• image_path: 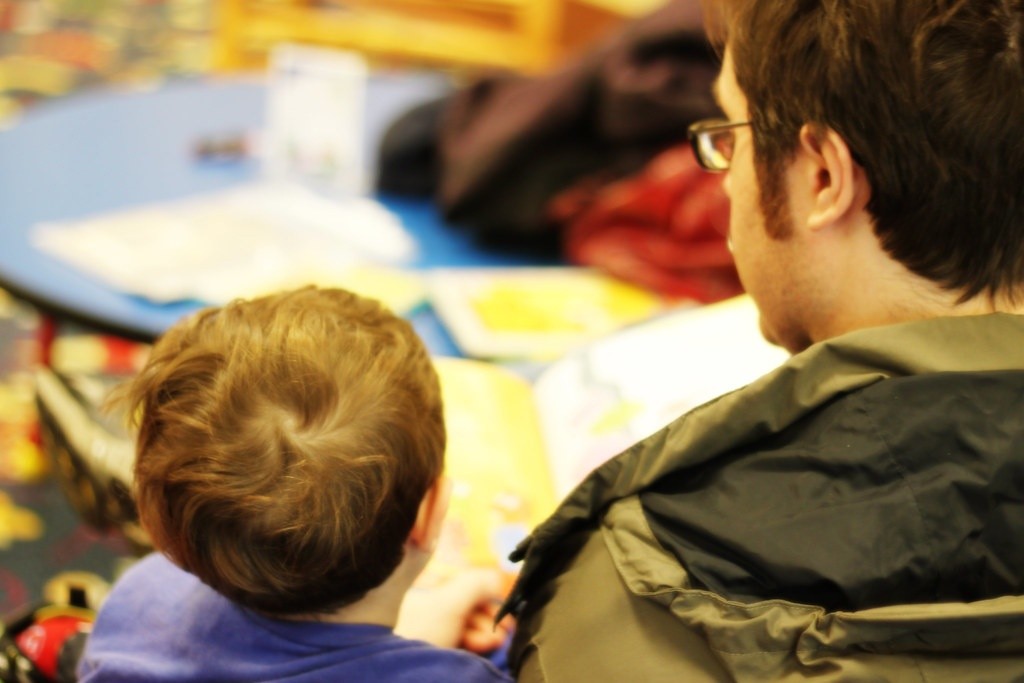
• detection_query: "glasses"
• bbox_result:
[688,117,751,172]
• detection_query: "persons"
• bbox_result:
[75,283,524,683]
[487,0,1024,683]
